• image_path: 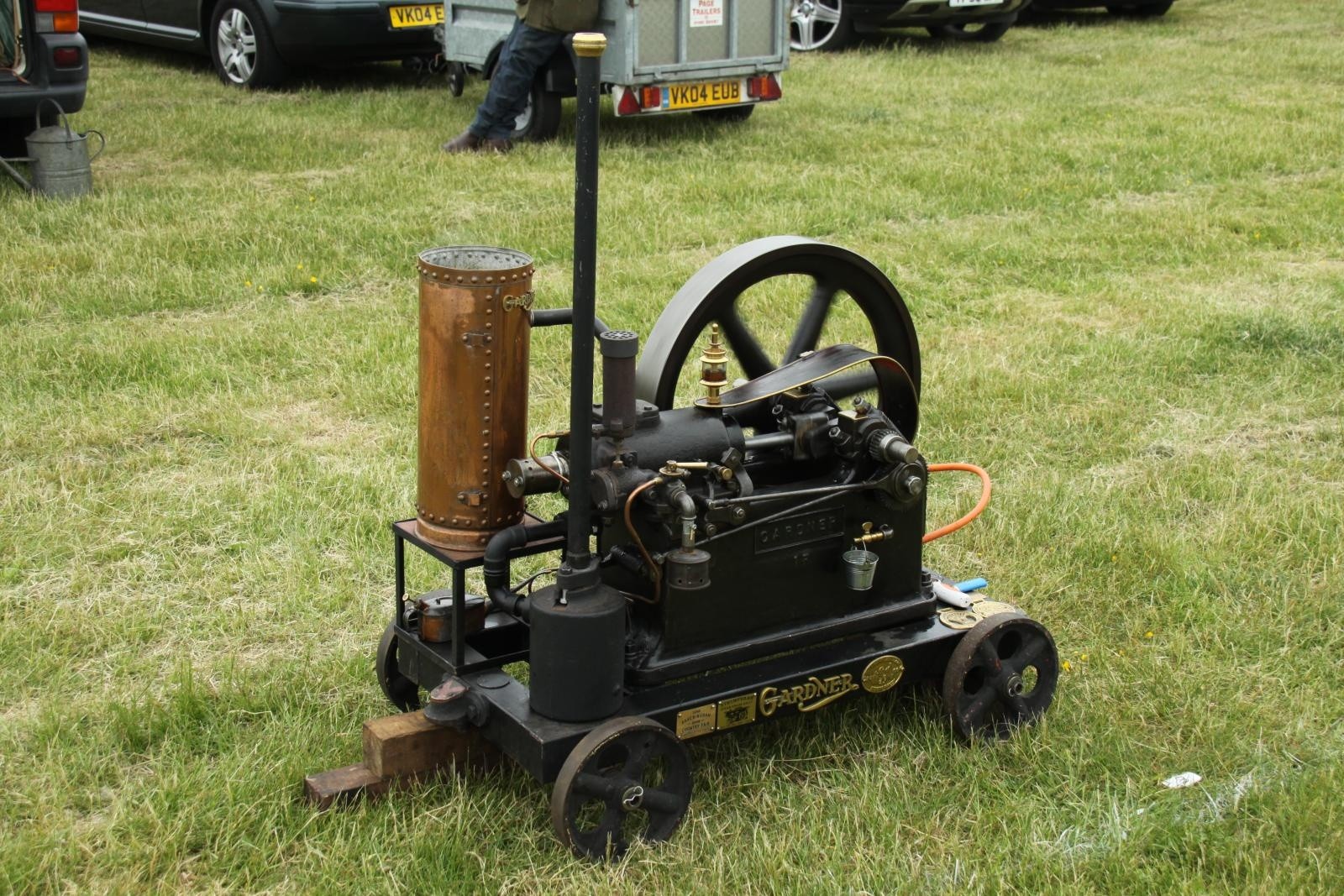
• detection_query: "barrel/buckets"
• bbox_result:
[842,537,879,591]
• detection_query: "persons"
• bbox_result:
[442,0,600,155]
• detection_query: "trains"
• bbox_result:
[374,26,1060,867]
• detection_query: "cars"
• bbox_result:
[0,0,89,154]
[78,0,449,92]
[789,0,1028,55]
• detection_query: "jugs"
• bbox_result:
[1,97,106,197]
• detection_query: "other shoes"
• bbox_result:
[482,137,513,152]
[444,131,478,152]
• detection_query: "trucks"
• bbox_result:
[445,0,792,126]
